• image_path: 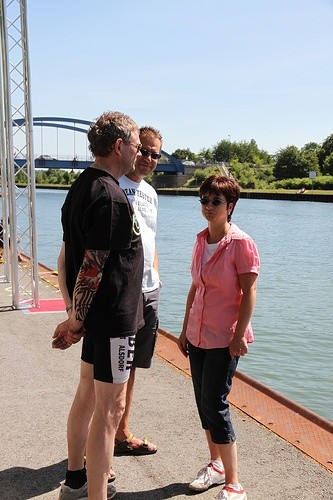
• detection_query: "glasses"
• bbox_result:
[127,140,142,152]
[140,148,161,159]
[199,198,228,206]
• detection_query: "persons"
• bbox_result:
[84,128,163,481]
[177,176,260,500]
[51,112,144,499]
[0,216,27,261]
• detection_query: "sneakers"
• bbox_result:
[58,483,116,500]
[189,463,225,491]
[215,483,247,500]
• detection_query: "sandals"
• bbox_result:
[84,456,116,481]
[114,432,157,456]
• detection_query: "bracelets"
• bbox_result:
[66,304,72,312]
